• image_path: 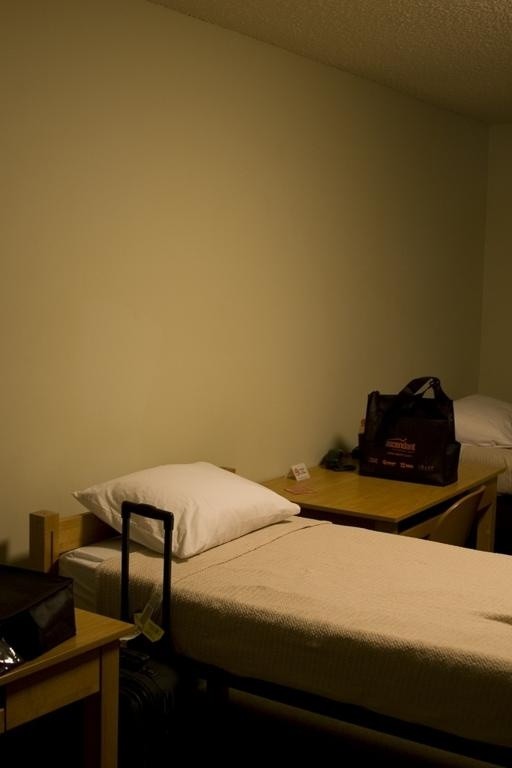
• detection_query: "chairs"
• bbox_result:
[431,485,486,547]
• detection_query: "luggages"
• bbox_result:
[74,501,193,768]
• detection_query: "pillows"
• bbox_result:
[453,394,511,448]
[74,458,301,559]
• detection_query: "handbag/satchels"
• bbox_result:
[358,377,461,487]
[0,564,76,677]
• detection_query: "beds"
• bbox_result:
[459,443,512,494]
[25,466,512,768]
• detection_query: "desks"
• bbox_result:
[261,460,507,554]
[0,601,136,766]
